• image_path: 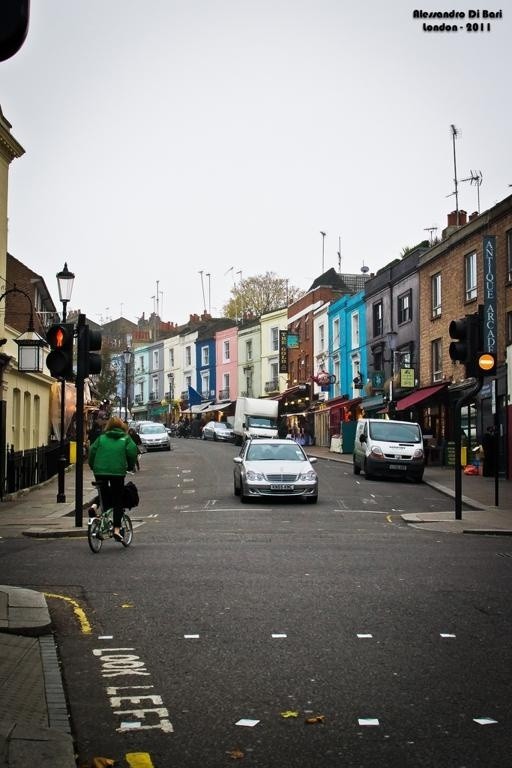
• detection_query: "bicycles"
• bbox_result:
[85,479,136,555]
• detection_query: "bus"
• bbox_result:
[201,420,239,443]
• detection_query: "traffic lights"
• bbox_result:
[44,319,77,383]
[445,315,473,365]
[82,323,106,381]
[476,351,501,378]
[387,400,399,417]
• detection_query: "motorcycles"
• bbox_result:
[170,420,202,439]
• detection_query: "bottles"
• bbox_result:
[106,515,113,529]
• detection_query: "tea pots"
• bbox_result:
[310,370,336,387]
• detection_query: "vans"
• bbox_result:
[353,418,429,483]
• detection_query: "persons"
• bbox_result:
[126,427,141,476]
[481,425,497,478]
[87,414,139,542]
[171,414,203,440]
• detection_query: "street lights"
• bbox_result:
[122,345,134,425]
[53,260,78,506]
[243,365,253,397]
[166,374,174,428]
[386,326,401,420]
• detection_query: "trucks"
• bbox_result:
[232,396,281,446]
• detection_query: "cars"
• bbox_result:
[111,405,175,452]
[230,436,324,510]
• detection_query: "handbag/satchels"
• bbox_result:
[124,482,139,508]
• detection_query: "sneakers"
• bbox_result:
[114,534,123,539]
[88,508,97,517]
